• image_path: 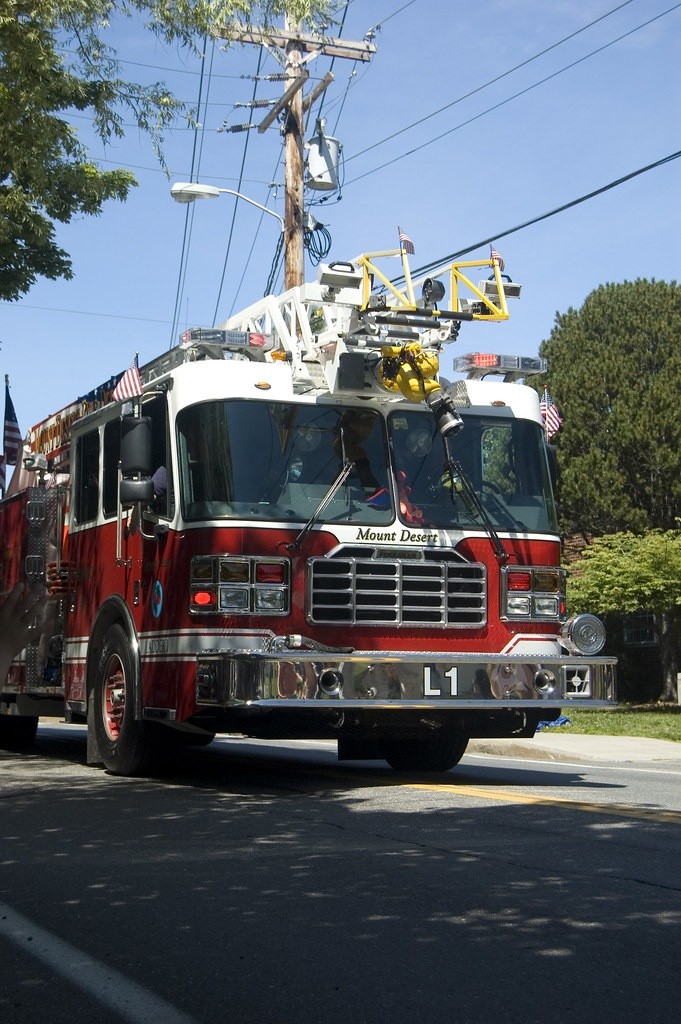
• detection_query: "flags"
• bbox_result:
[107,356,149,402]
[539,390,563,438]
[0,455,6,489]
[2,380,23,465]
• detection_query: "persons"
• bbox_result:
[0,581,55,693]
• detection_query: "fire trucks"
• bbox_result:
[2,229,608,778]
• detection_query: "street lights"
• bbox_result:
[170,182,305,292]
